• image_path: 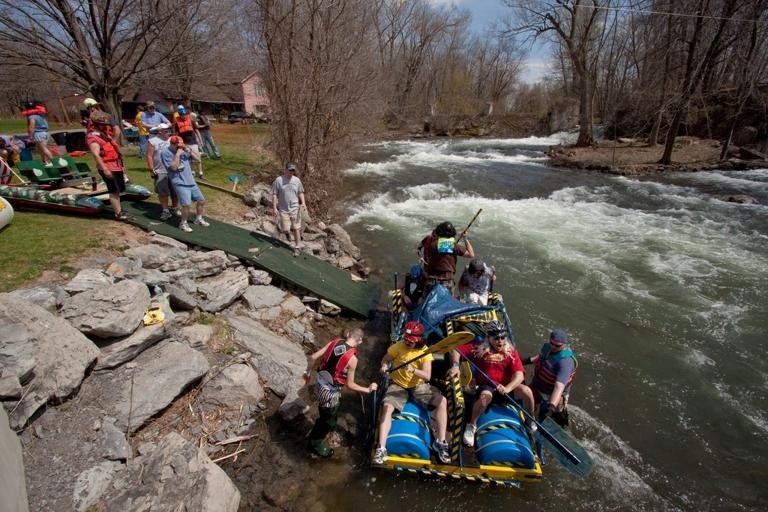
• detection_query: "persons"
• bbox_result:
[79,98,120,142]
[86,111,135,220]
[306,327,377,457]
[459,260,496,306]
[400,264,426,310]
[417,228,435,270]
[172,106,197,122]
[161,137,210,232]
[135,106,148,158]
[25,103,54,166]
[174,108,204,179]
[421,222,475,304]
[141,101,172,138]
[372,322,451,465]
[197,109,221,158]
[147,124,182,220]
[522,328,578,430]
[444,321,536,447]
[272,163,306,255]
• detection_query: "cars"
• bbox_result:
[228,111,248,124]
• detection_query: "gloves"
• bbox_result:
[519,356,532,365]
[539,403,554,421]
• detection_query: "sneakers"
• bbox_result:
[179,219,192,233]
[199,174,206,179]
[116,211,130,220]
[433,438,451,464]
[464,424,478,447]
[215,154,221,158]
[194,219,210,227]
[171,207,182,215]
[159,210,172,221]
[42,160,53,167]
[294,246,300,258]
[527,416,538,430]
[372,446,390,464]
[287,241,296,249]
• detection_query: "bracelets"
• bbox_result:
[412,368,416,374]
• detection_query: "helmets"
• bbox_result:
[177,105,184,109]
[409,265,422,277]
[486,320,508,338]
[179,109,186,114]
[401,322,425,344]
[92,111,116,125]
[436,221,457,238]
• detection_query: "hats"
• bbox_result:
[156,124,170,134]
[286,162,297,170]
[80,98,103,109]
[197,109,204,114]
[25,101,33,108]
[469,258,485,273]
[171,136,184,146]
[144,101,156,109]
[551,329,568,346]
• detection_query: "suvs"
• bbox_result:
[80,101,170,126]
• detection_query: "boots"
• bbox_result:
[308,422,336,457]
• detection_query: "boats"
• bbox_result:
[371,288,545,487]
[0,158,152,213]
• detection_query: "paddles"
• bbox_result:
[230,175,241,192]
[389,331,474,374]
[420,316,594,478]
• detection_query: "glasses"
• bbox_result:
[492,336,506,342]
[550,341,565,348]
